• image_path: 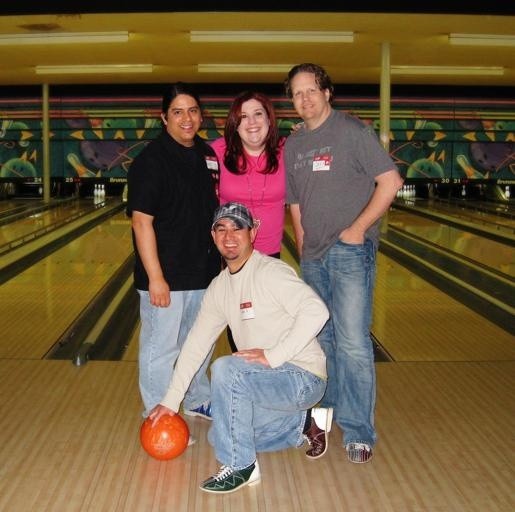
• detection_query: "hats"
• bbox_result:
[211,202,253,230]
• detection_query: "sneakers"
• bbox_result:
[184,399,212,420]
[199,459,261,494]
[187,436,197,446]
[347,443,374,464]
[302,407,333,459]
